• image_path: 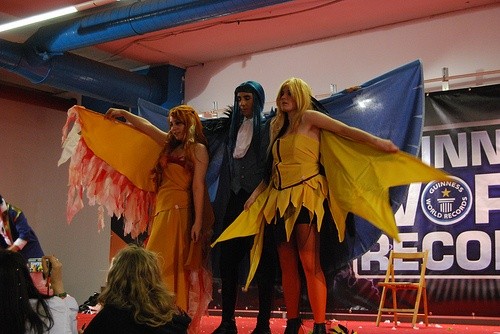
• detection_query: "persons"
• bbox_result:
[211,78,400,334]
[0,195,45,263]
[103,105,213,334]
[203,80,362,334]
[78,243,192,334]
[0,249,80,334]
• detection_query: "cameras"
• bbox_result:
[27,258,51,272]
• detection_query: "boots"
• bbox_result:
[212,277,238,334]
[251,288,274,334]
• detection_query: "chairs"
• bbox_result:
[375,250,430,328]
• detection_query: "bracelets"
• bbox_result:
[12,245,20,251]
[54,292,66,297]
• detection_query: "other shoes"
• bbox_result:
[284,317,307,334]
[313,323,326,334]
